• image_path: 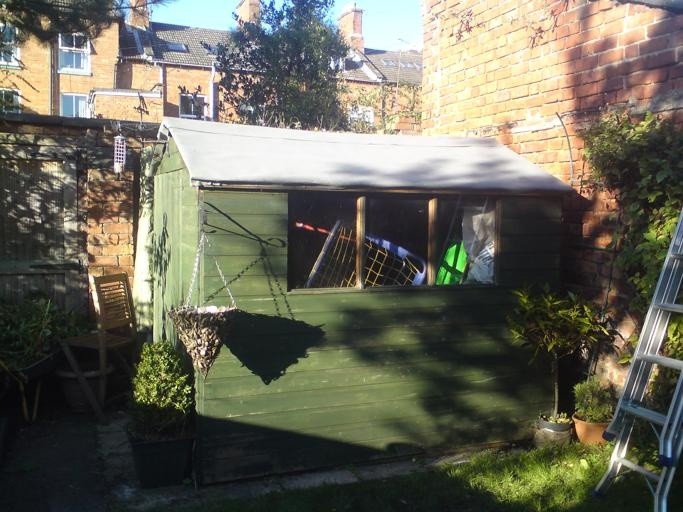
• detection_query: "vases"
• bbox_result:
[58,365,108,417]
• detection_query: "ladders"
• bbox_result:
[590,204,683,511]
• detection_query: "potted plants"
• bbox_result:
[505,280,618,449]
[124,338,196,490]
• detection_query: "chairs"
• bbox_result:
[60,270,141,419]
